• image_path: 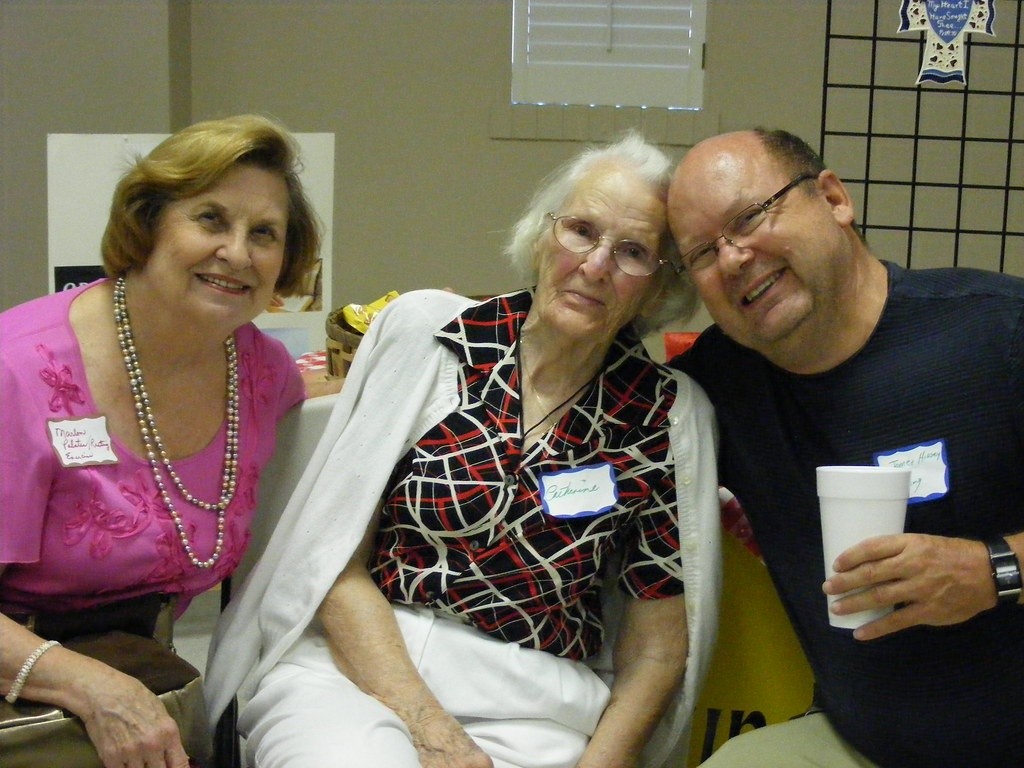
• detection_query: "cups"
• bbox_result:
[815,463,913,631]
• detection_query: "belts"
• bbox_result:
[4,595,170,642]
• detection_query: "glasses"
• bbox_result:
[548,212,673,277]
[673,171,817,275]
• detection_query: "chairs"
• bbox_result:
[220,394,350,767]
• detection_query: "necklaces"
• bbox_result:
[115,274,239,567]
[517,329,599,448]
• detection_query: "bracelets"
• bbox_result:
[4,640,62,704]
[981,535,1022,611]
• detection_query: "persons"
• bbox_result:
[0,114,328,768]
[202,134,716,768]
[662,125,1024,768]
